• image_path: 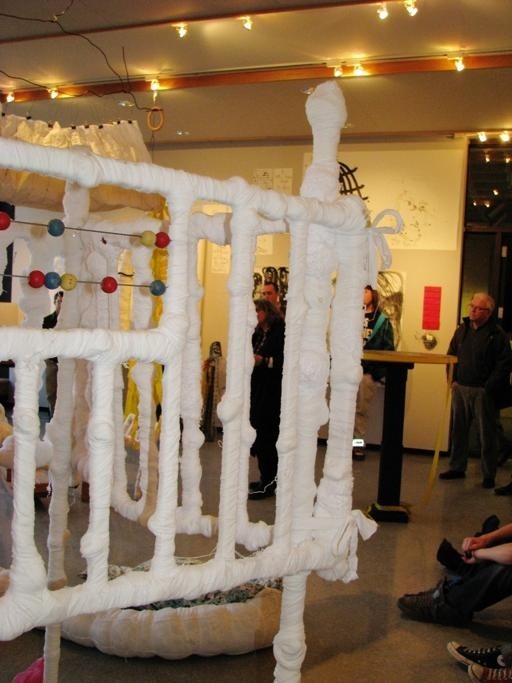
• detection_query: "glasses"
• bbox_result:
[469,304,489,311]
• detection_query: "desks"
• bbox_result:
[352,350,459,524]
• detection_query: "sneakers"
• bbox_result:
[467,663,512,683]
[397,576,473,625]
[447,640,506,668]
[482,478,494,488]
[439,469,465,479]
[495,482,512,496]
[248,481,276,499]
[353,447,365,460]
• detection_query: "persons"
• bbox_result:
[41,291,64,410]
[353,284,396,459]
[396,522,512,627]
[437,291,511,489]
[446,639,512,682]
[247,298,286,501]
[255,282,286,322]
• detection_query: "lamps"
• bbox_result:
[6,0,465,103]
